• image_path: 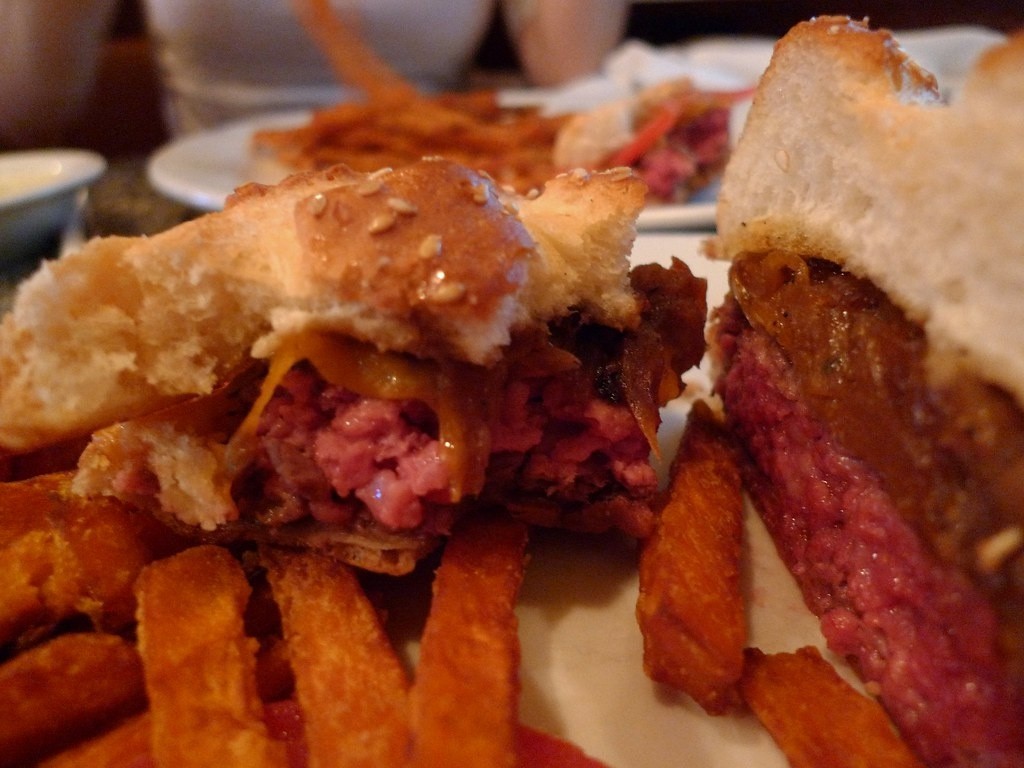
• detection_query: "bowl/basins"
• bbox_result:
[0,147,109,262]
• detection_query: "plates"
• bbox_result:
[395,232,879,768]
[148,85,754,232]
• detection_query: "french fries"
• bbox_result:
[248,0,569,195]
[0,410,945,768]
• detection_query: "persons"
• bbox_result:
[0,0,632,154]
